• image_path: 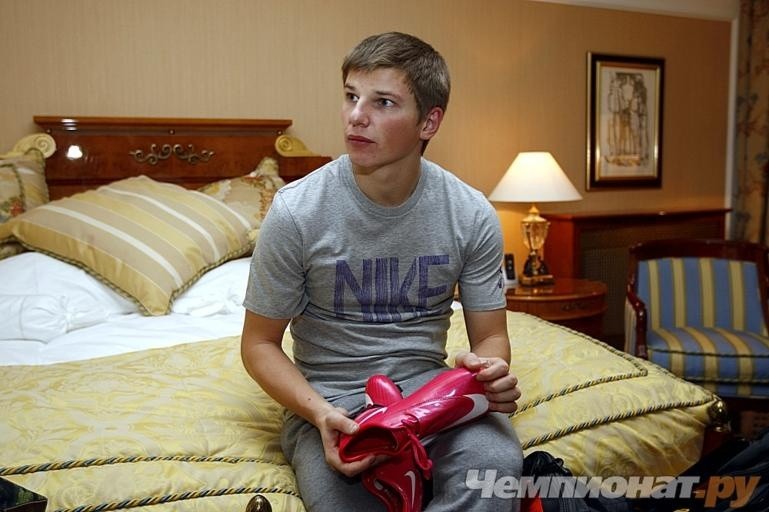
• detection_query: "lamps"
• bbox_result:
[488,152,583,288]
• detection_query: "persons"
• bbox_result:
[237,30,525,511]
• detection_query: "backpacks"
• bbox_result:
[520,451,572,512]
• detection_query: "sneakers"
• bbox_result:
[339,368,491,512]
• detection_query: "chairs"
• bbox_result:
[621,239,769,437]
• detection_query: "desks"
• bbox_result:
[539,208,734,338]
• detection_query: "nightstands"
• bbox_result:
[501,277,606,345]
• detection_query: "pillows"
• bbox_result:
[195,156,287,252]
[11,174,255,317]
[0,253,140,345]
[0,148,49,263]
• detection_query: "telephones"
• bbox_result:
[501,253,519,286]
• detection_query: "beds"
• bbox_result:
[0,115,733,512]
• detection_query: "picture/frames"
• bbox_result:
[587,51,666,190]
